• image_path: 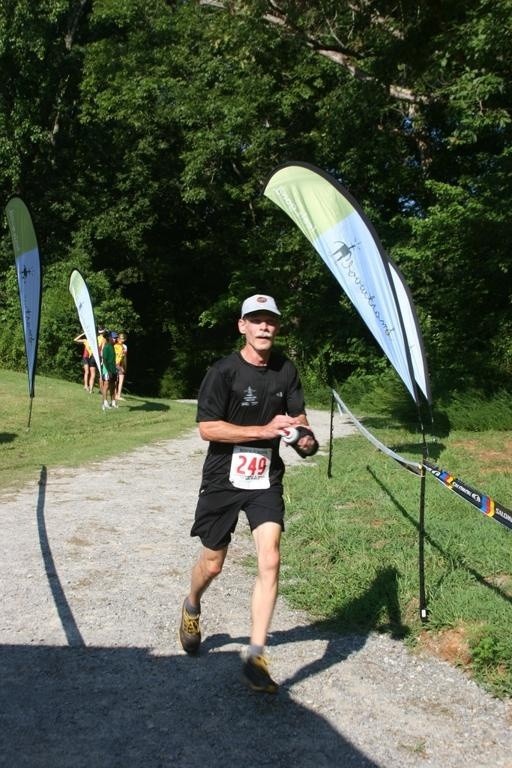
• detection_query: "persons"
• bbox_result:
[74,326,129,409]
[179,294,319,693]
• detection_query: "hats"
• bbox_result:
[241,294,281,316]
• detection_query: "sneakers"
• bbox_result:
[178,598,200,653]
[241,655,278,693]
[103,404,118,409]
